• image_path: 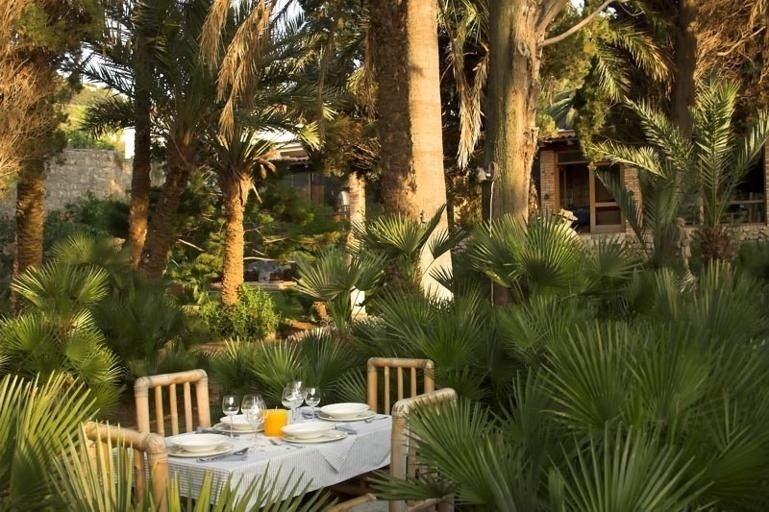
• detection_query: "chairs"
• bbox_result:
[364,356,434,420]
[133,368,211,441]
[332,386,461,510]
[81,420,177,512]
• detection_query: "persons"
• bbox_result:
[670,216,692,276]
[548,208,580,241]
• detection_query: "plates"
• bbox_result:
[281,430,348,444]
[161,414,265,457]
[320,410,377,422]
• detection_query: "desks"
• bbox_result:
[107,407,412,512]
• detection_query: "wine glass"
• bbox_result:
[222,394,267,456]
[281,380,322,423]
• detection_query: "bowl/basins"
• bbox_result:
[321,403,369,418]
[282,422,335,438]
